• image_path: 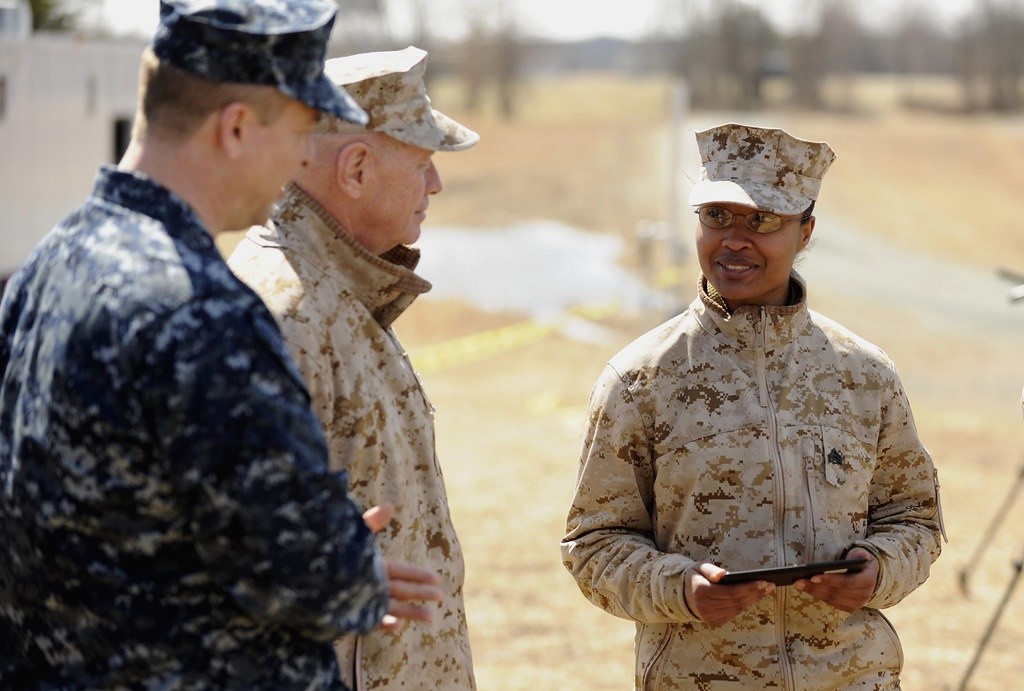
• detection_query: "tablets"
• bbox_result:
[717,558,869,590]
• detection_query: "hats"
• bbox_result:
[325,45,481,151]
[689,123,837,216]
[152,0,371,126]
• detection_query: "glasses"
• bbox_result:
[695,205,813,234]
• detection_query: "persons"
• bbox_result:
[223,46,481,691]
[0,0,443,691]
[559,124,947,691]
